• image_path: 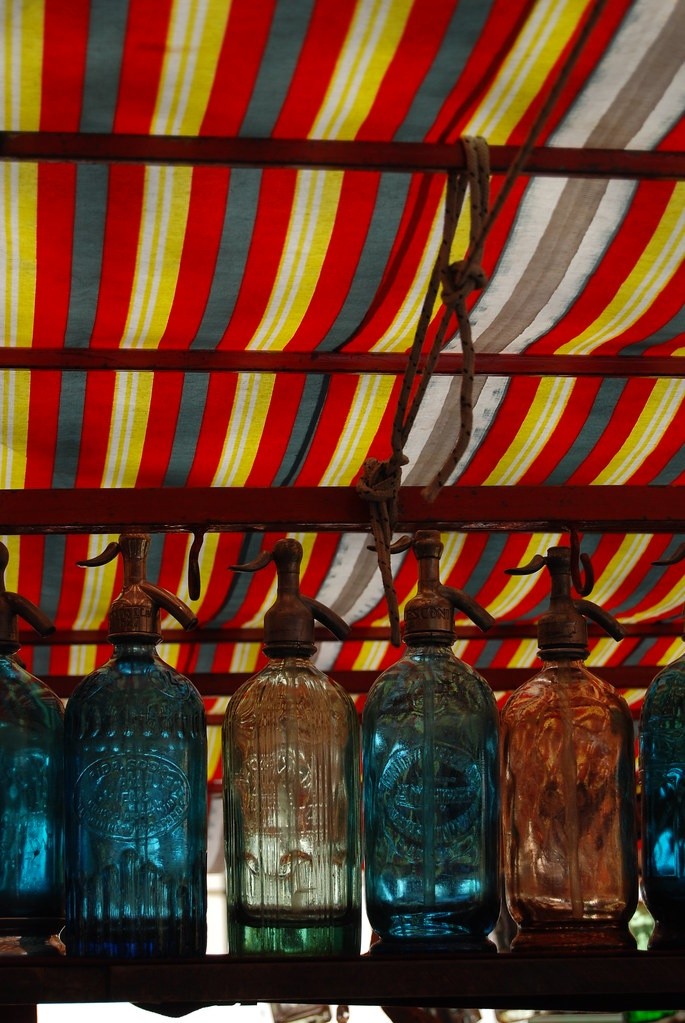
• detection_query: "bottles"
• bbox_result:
[503,649,634,926]
[64,638,208,924]
[221,645,360,922]
[354,631,503,939]
[0,639,64,917]
[640,631,685,923]
